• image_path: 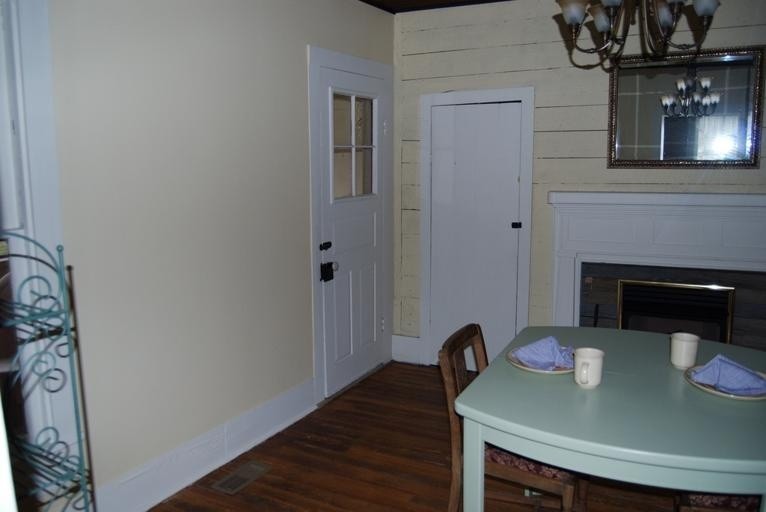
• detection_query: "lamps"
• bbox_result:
[553,0,721,72]
[658,65,719,117]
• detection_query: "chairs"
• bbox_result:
[439,322,589,512]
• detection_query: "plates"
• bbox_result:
[506,346,574,376]
[684,362,765,400]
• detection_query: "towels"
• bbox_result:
[512,335,573,370]
[690,355,766,394]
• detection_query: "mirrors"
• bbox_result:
[607,44,766,168]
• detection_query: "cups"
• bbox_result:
[572,347,606,390]
[669,330,700,372]
